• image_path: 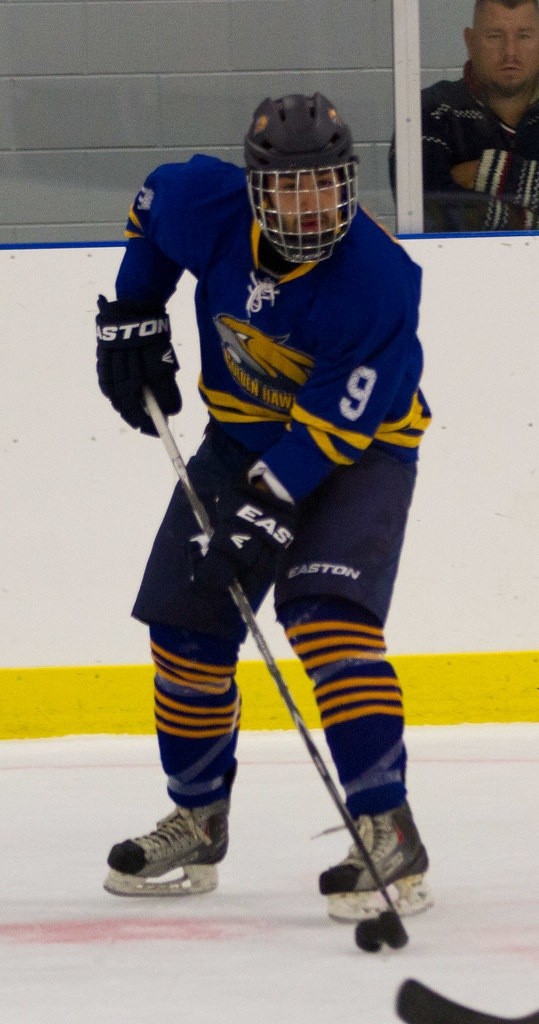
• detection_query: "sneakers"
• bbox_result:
[320,798,432,919]
[102,794,231,893]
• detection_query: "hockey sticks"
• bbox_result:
[96,293,410,952]
[393,977,539,1024]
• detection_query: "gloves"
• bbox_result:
[96,295,183,437]
[187,482,302,590]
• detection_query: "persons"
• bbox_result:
[386,1,539,233]
[95,92,435,924]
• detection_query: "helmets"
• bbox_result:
[243,94,359,264]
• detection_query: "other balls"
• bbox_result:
[355,919,384,951]
